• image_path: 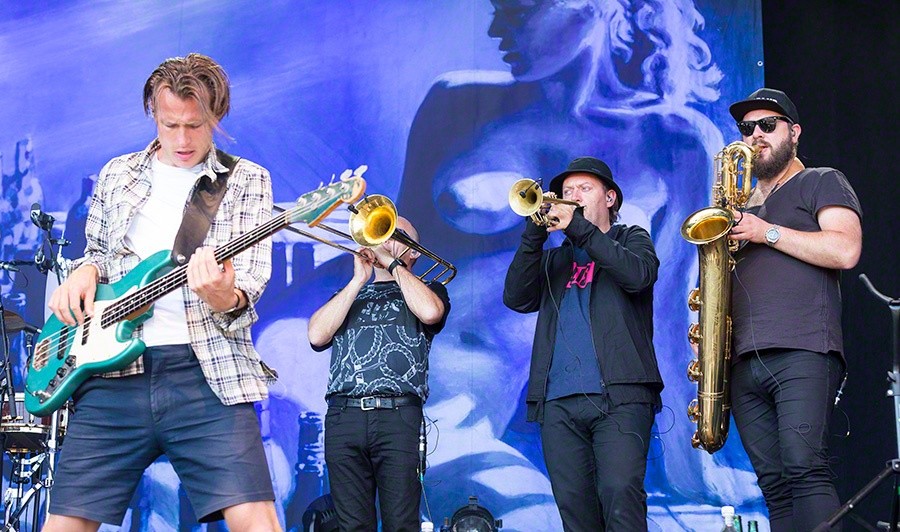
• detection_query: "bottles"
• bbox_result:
[282,411,330,532]
[720,504,759,532]
[441,517,451,532]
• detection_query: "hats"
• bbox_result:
[549,157,623,213]
[728,88,799,124]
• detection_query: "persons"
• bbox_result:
[502,157,665,532]
[41,52,285,532]
[307,214,452,532]
[716,87,862,532]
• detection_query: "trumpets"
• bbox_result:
[509,177,584,228]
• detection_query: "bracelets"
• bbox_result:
[222,293,240,314]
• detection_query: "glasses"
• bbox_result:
[736,116,795,137]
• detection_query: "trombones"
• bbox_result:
[270,192,458,288]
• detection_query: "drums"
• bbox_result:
[0,391,72,449]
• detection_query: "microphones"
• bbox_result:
[348,205,358,214]
[520,178,543,199]
[0,261,20,272]
[721,197,743,226]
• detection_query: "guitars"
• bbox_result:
[23,161,370,420]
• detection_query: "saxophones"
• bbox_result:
[685,139,765,455]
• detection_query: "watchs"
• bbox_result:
[387,258,408,276]
[764,223,782,248]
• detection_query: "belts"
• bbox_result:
[329,396,421,410]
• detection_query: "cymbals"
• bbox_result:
[1,310,25,335]
